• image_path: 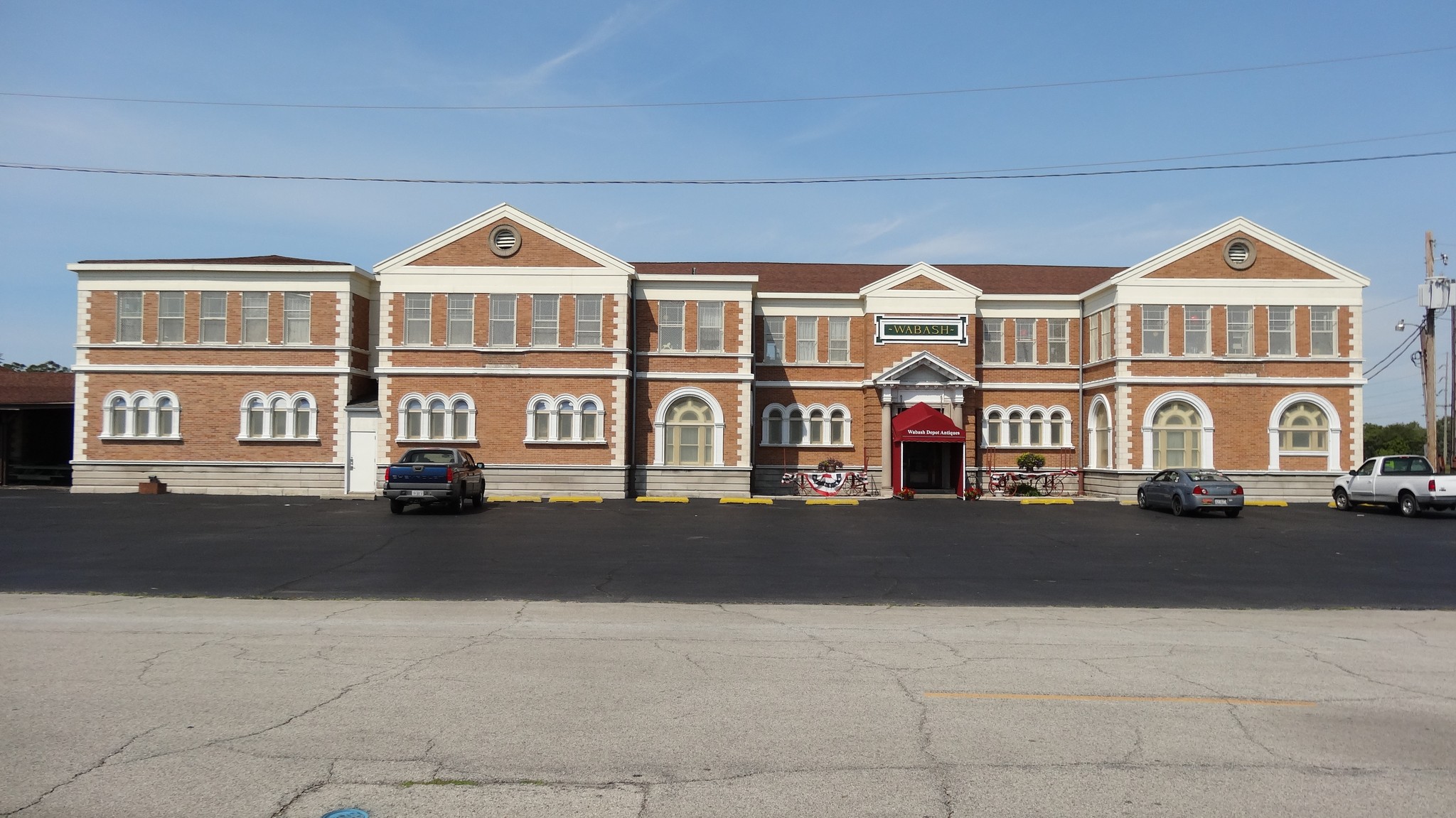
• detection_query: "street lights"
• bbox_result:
[1396,320,1439,472]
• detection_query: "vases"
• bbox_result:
[903,496,909,501]
[969,496,975,502]
[994,491,1005,496]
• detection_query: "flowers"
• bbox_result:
[898,487,917,496]
[964,487,984,498]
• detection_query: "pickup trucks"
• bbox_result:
[1330,455,1456,518]
[383,449,486,514]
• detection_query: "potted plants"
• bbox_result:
[140,476,167,494]
[1016,452,1046,473]
[816,457,844,472]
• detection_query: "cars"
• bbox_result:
[1137,468,1245,517]
